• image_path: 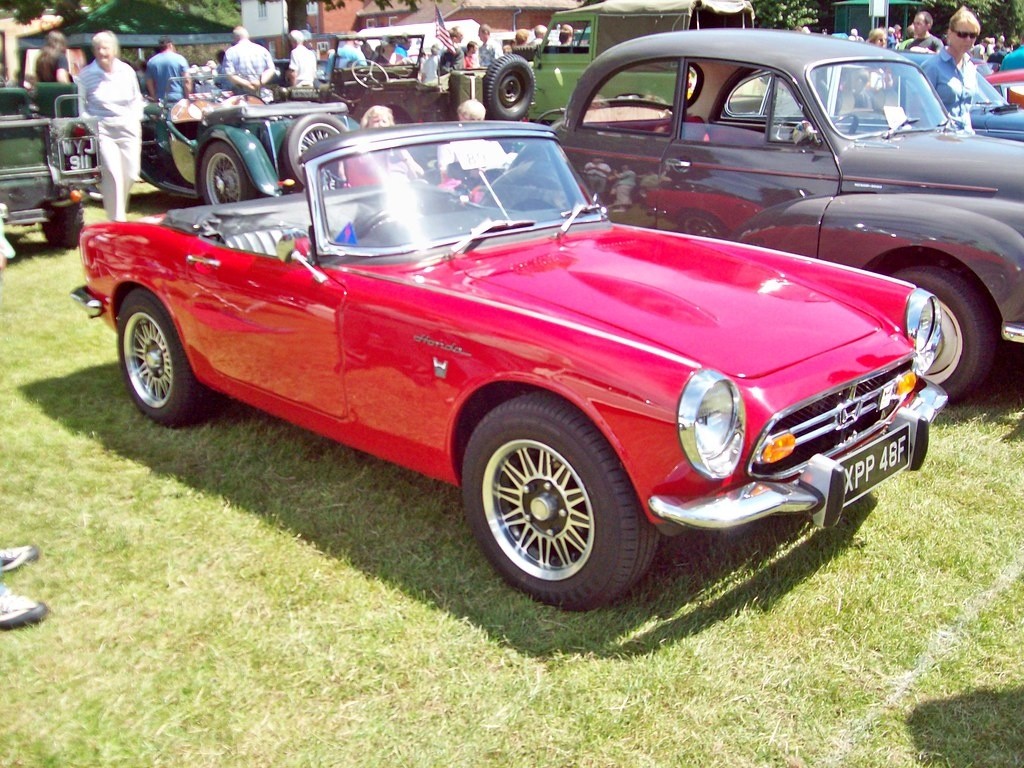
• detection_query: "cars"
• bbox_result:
[901,54,1024,140]
[555,28,1024,403]
[319,55,536,121]
[140,73,349,205]
[0,88,85,250]
[69,121,948,612]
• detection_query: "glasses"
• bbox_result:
[878,39,883,42]
[951,29,978,39]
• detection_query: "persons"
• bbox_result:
[346,105,426,188]
[139,22,575,104]
[0,543,46,631]
[33,29,72,84]
[579,156,677,213]
[76,30,145,225]
[436,97,518,204]
[795,6,1024,139]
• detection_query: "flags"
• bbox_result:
[435,4,456,54]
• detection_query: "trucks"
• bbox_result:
[535,1,756,119]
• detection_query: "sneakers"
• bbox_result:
[0,544,39,573]
[0,584,49,632]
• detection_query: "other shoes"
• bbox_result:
[613,208,627,213]
